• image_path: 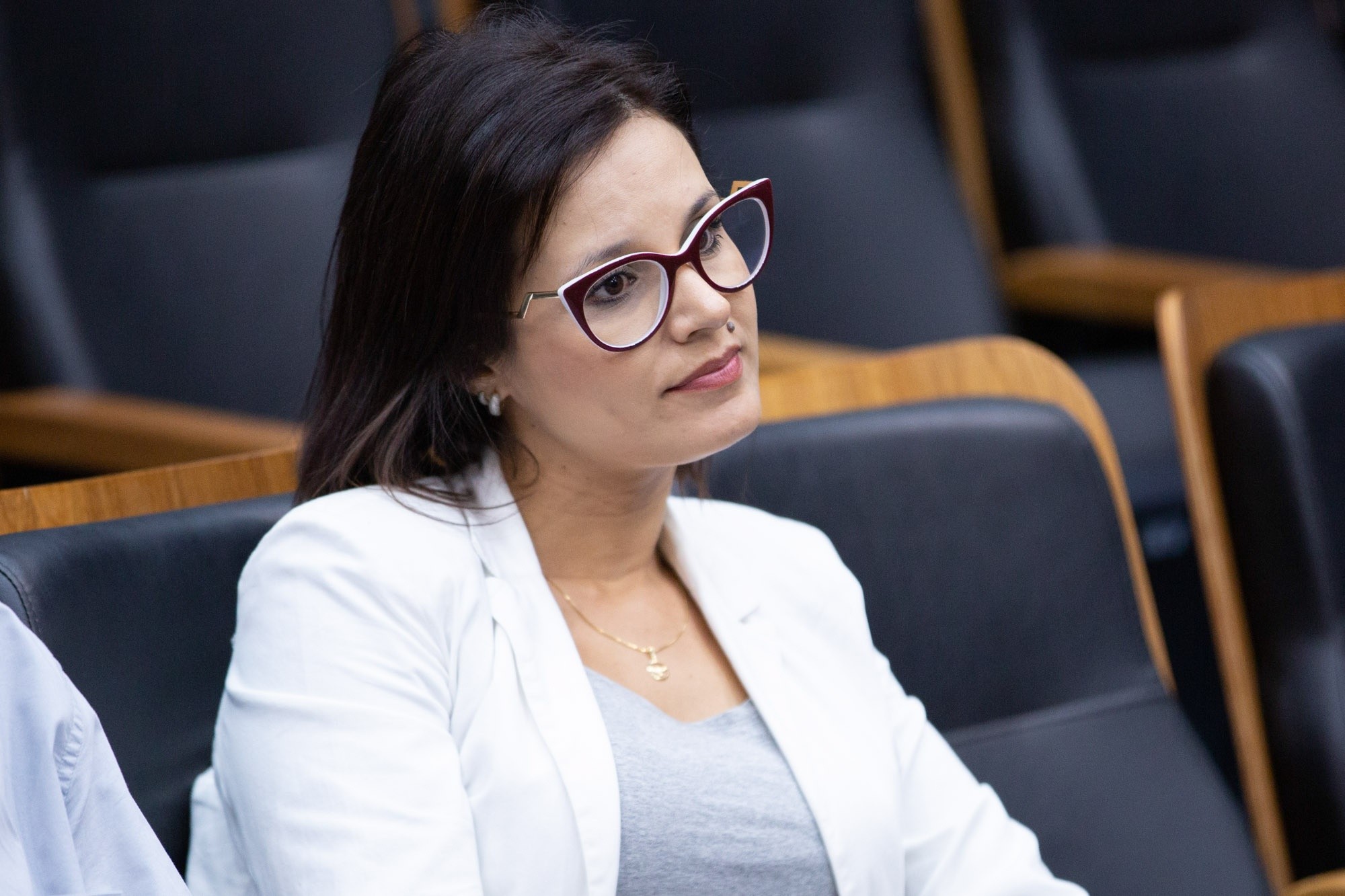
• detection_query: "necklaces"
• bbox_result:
[545,576,689,681]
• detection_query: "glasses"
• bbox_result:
[506,178,775,352]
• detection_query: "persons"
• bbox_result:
[0,604,192,896]
[187,17,1090,896]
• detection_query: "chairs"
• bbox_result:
[0,0,1345,896]
